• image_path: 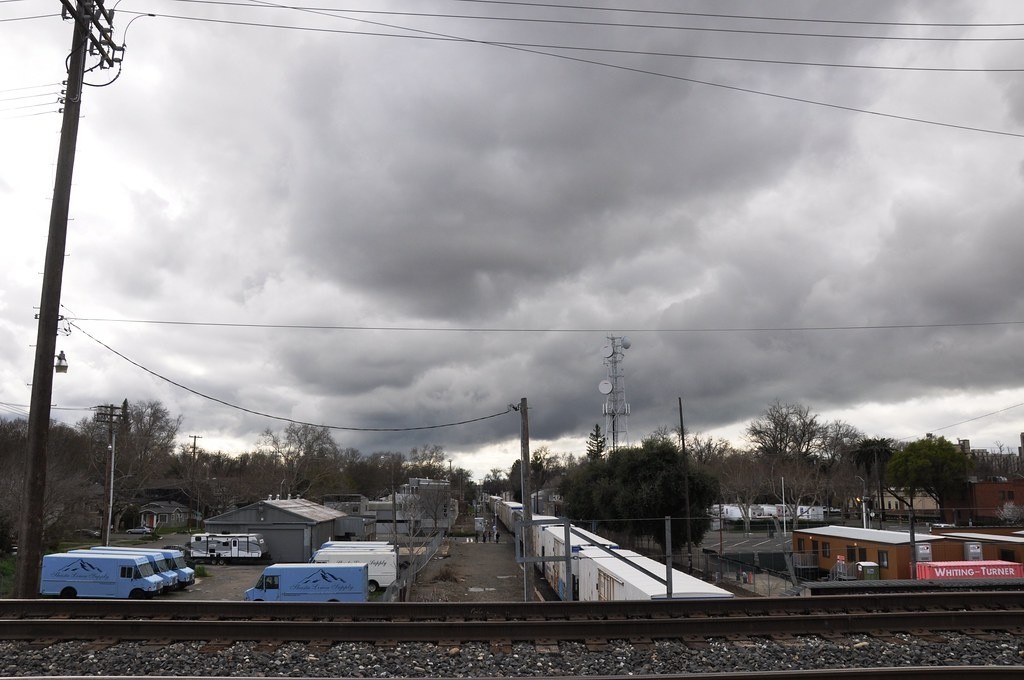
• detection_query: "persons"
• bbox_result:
[488,531,492,543]
[475,530,480,543]
[492,524,500,543]
[482,531,487,543]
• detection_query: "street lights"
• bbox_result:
[105,436,116,545]
[101,474,134,546]
[195,477,217,532]
[18,350,68,598]
[448,458,453,499]
[380,454,399,583]
[494,501,500,528]
[854,476,867,528]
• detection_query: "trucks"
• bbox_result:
[38,552,164,600]
[307,540,400,593]
[67,548,180,595]
[90,546,196,591]
[243,562,370,601]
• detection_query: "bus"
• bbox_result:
[189,531,270,565]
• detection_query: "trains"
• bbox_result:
[488,495,736,602]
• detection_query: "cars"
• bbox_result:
[126,526,152,535]
[71,529,103,540]
